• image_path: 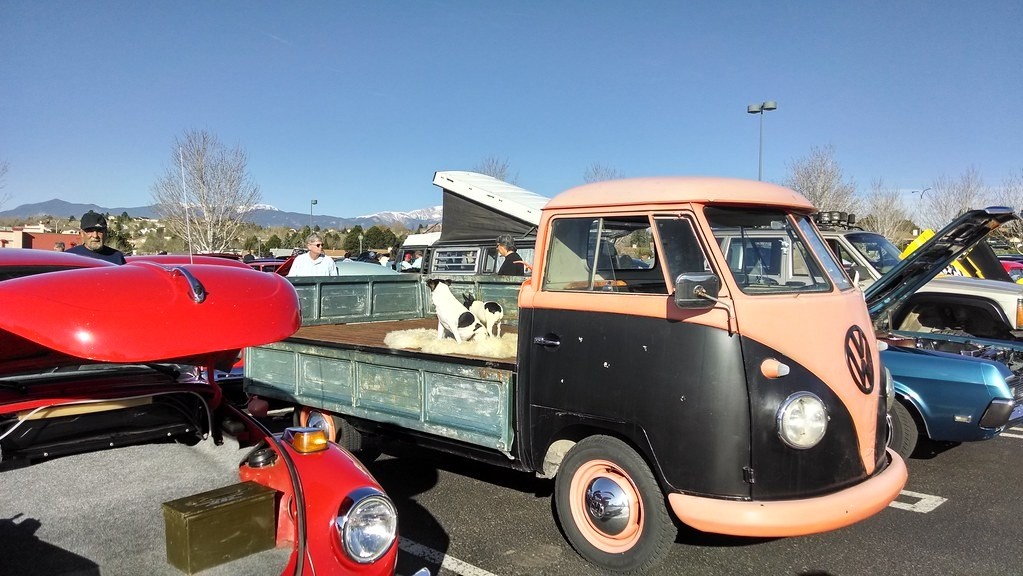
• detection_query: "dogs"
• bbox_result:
[426,279,503,342]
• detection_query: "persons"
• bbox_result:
[286,234,337,276]
[400,251,422,270]
[53,211,127,264]
[496,234,524,275]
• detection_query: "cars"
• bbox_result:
[898,229,1023,286]
[1,250,400,576]
[861,206,1023,461]
[121,256,250,390]
[246,261,285,273]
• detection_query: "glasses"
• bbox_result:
[310,244,323,247]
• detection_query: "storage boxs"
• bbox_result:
[162,480,277,576]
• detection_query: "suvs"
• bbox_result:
[709,212,1023,348]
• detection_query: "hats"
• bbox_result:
[406,254,412,258]
[415,251,423,256]
[80,210,107,231]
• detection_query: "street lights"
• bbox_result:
[311,199,317,234]
[748,103,778,179]
[912,186,933,236]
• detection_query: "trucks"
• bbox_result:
[393,171,537,276]
[241,175,909,575]
